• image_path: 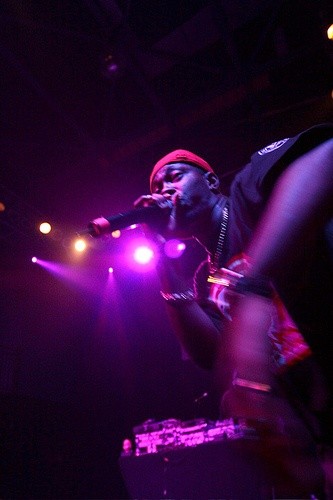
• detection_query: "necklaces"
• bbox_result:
[206,206,232,277]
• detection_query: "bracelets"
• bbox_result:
[158,288,199,303]
[218,265,274,302]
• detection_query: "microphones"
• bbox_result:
[194,393,208,402]
[88,201,177,239]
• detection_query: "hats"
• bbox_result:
[149,149,214,195]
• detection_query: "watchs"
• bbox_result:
[140,122,332,500]
